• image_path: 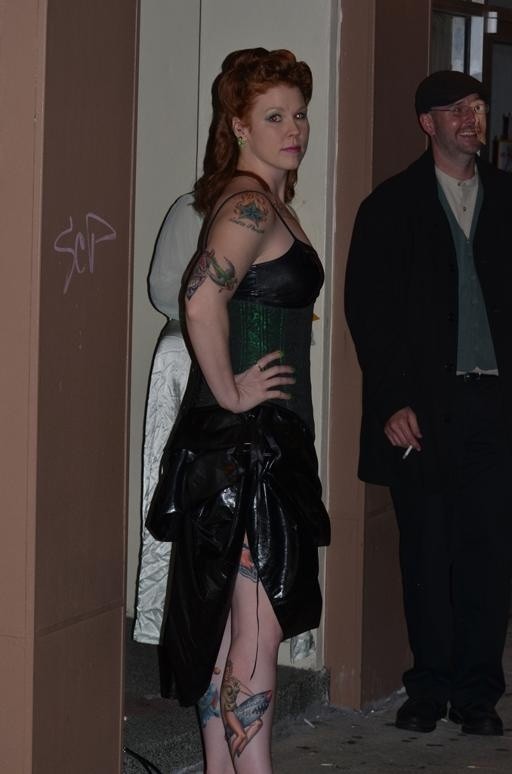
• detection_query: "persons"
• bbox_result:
[146,47,332,773]
[345,69,512,736]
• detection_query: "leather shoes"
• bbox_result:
[395,697,448,733]
[447,701,504,735]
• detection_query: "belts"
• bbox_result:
[456,373,496,383]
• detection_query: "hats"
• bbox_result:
[414,70,487,115]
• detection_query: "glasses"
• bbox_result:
[429,102,490,117]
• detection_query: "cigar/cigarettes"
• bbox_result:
[402,445,413,460]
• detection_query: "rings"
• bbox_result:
[255,363,262,372]
